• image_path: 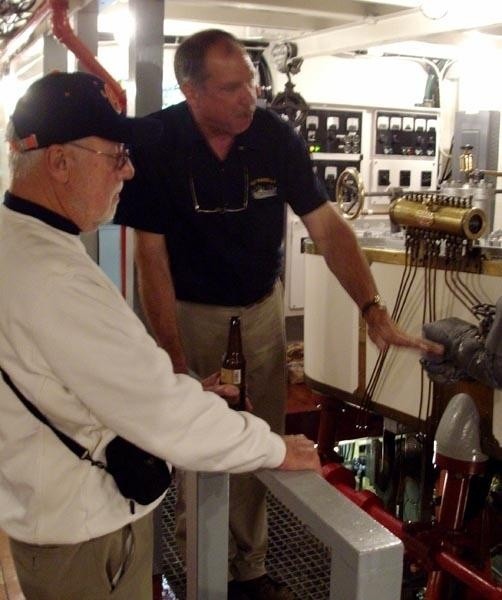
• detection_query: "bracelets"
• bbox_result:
[361,294,386,318]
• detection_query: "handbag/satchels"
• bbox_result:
[104,434,171,505]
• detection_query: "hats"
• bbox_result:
[10,70,163,154]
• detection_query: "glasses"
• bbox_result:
[67,141,129,170]
[188,168,249,215]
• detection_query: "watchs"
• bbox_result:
[111,27,446,600]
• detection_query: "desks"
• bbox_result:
[301,238,502,447]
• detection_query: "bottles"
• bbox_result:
[219,315,246,411]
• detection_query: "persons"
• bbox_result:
[1,64,323,600]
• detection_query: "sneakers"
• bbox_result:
[228,574,295,600]
